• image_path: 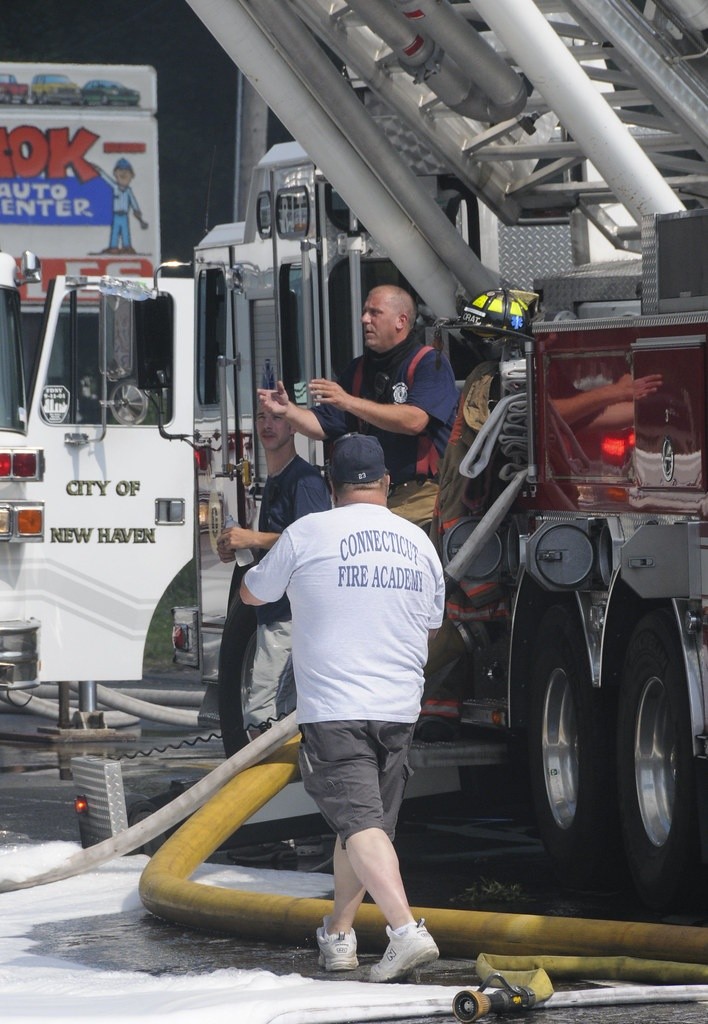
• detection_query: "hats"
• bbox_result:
[330,432,385,484]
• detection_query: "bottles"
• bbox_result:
[224,515,254,567]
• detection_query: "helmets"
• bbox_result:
[459,287,532,338]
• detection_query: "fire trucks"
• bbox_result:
[2,0,708,906]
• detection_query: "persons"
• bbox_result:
[416,337,662,744]
[216,390,446,983]
[253,284,463,528]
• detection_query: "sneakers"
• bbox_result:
[370,918,440,983]
[315,915,359,971]
